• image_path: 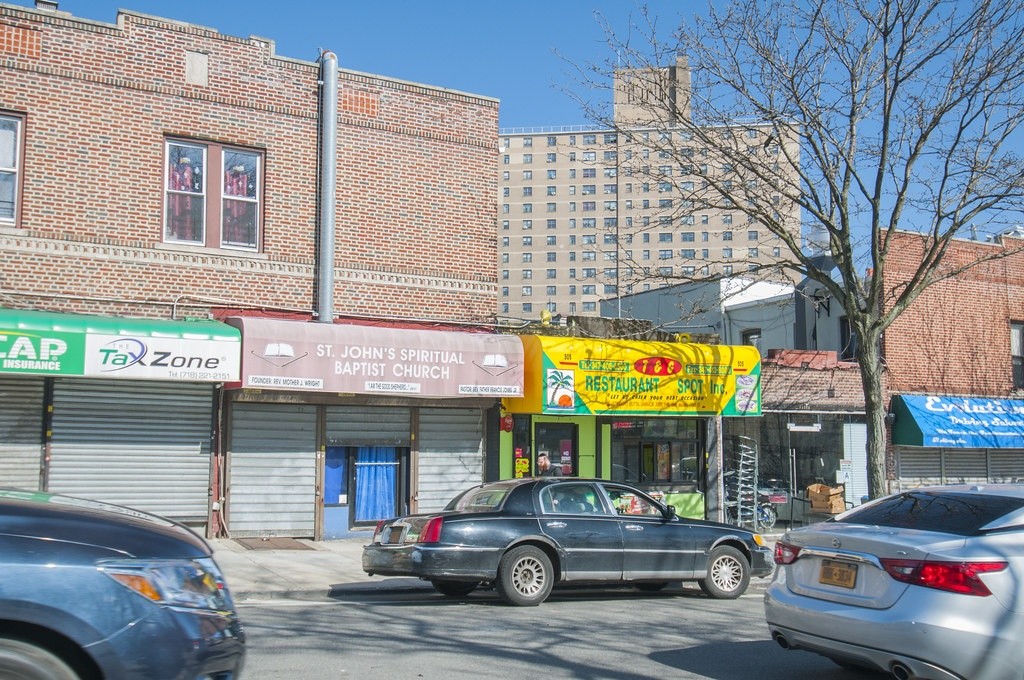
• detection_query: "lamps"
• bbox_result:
[540,310,552,324]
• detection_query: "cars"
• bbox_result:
[551,463,648,483]
[765,484,1024,680]
[362,477,775,607]
[0,486,246,680]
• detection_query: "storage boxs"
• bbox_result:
[806,483,846,514]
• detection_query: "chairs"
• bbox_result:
[566,503,596,515]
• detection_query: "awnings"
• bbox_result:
[224,315,525,399]
[0,308,241,383]
[891,395,1024,451]
[507,333,763,417]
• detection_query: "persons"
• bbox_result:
[536,454,565,477]
